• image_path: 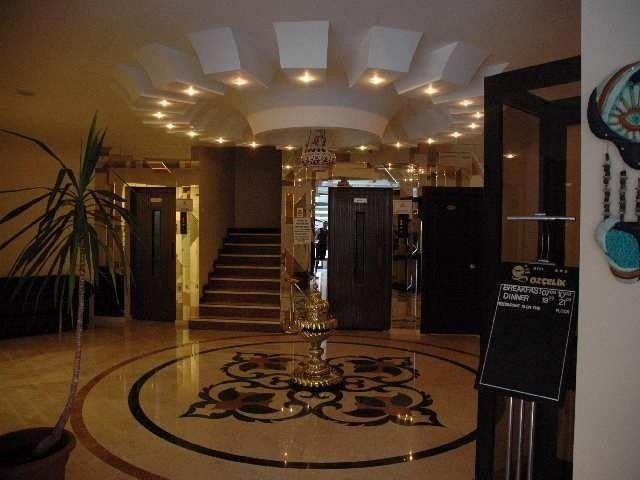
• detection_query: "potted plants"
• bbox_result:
[0,110,139,480]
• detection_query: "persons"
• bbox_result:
[314,221,328,268]
[405,218,419,291]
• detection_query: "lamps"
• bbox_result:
[300,126,338,174]
